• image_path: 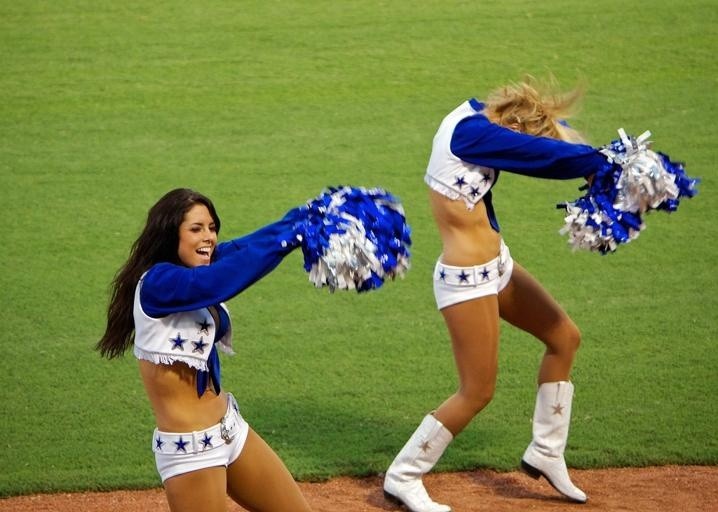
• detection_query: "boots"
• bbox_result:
[383,411,452,512]
[521,379,588,502]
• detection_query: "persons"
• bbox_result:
[94,187,326,511]
[381,82,615,512]
